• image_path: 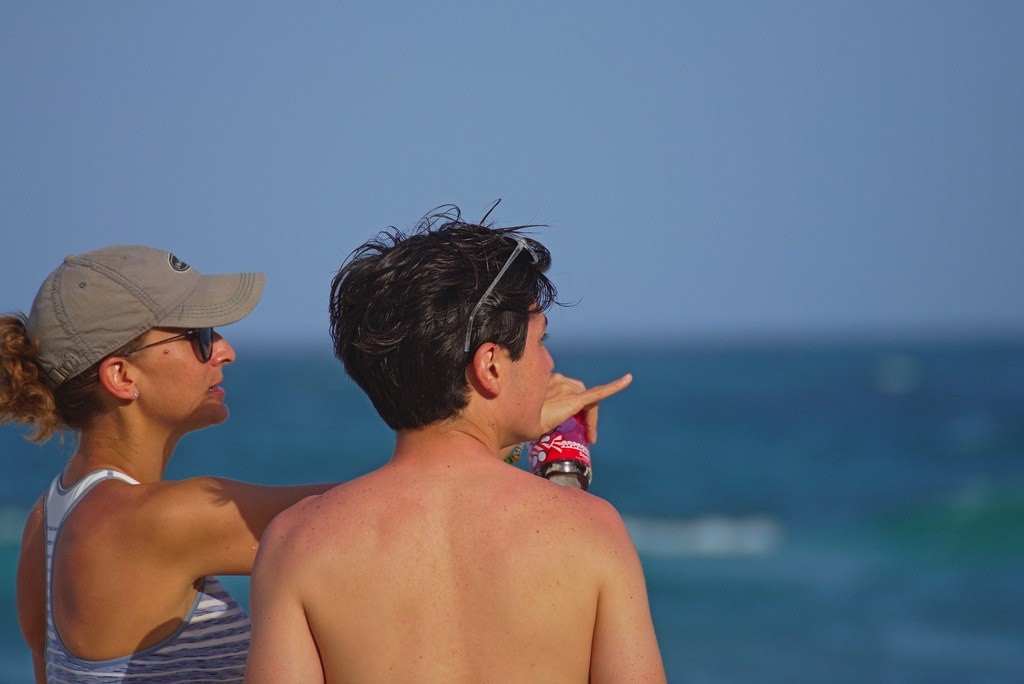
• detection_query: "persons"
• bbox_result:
[245,202,671,684]
[0,245,632,684]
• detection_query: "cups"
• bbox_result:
[526,407,593,494]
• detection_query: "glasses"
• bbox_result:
[464,232,539,355]
[119,327,216,363]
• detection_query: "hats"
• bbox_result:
[24,244,266,388]
